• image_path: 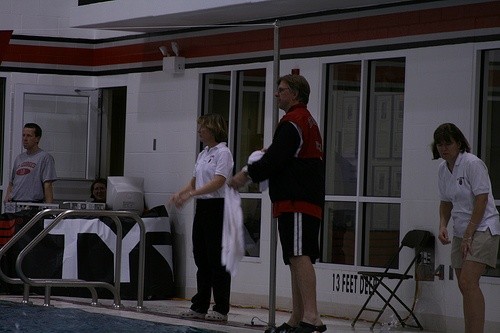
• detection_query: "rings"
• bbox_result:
[469,237,472,239]
[464,239,466,241]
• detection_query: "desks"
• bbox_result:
[0,204,173,301]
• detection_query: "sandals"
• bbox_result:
[179,309,205,319]
[206,310,226,321]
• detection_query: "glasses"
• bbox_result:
[278,87,289,93]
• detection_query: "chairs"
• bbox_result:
[351,230,433,331]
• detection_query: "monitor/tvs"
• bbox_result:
[106,176,145,217]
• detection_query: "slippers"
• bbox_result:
[270,322,299,333]
[289,322,327,333]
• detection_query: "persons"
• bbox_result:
[232,74,327,333]
[168,113,235,323]
[431,123,500,333]
[4,122,55,296]
[77,177,116,281]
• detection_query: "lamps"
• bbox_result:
[159,41,185,74]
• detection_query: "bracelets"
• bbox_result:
[189,191,193,196]
[470,222,479,226]
